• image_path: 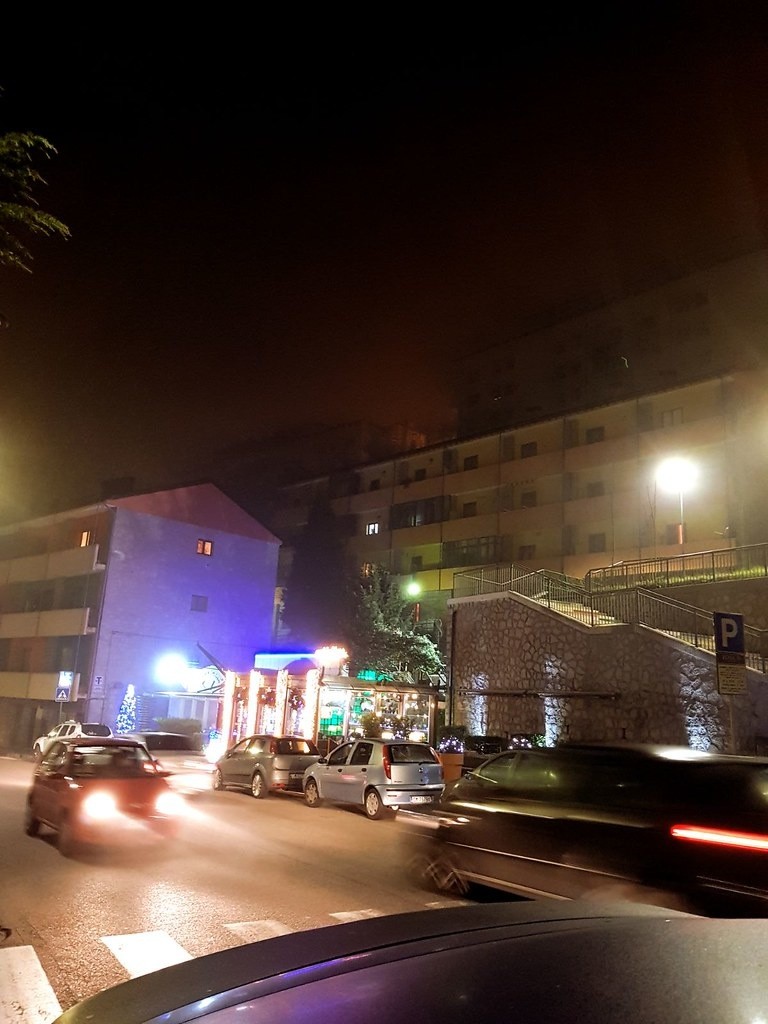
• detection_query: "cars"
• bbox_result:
[124,731,218,791]
[439,734,524,777]
[212,735,322,798]
[310,738,352,757]
[301,739,444,819]
[25,737,188,859]
[47,895,767,1023]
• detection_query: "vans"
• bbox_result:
[395,737,767,922]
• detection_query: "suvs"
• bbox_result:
[31,719,114,765]
